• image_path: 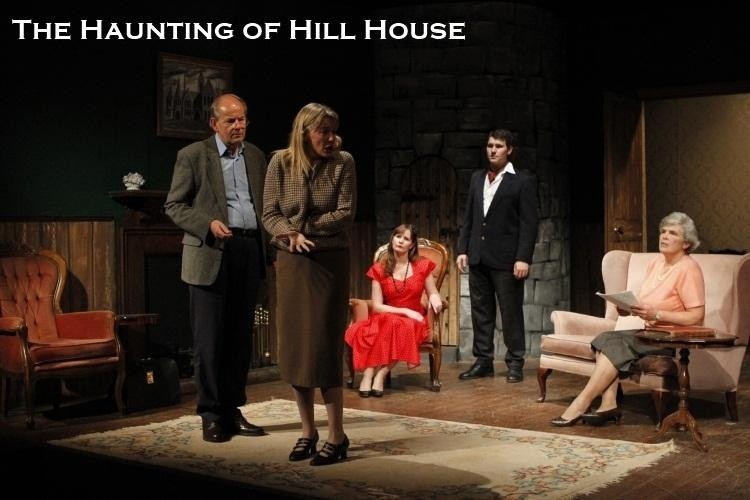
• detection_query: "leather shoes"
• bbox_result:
[201,418,228,442]
[506,370,523,382]
[459,364,494,379]
[231,410,265,436]
[358,390,383,398]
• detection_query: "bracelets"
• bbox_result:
[655,310,662,321]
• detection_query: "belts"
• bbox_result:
[228,228,254,237]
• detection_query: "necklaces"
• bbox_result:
[391,260,410,293]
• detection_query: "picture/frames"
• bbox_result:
[156,52,234,140]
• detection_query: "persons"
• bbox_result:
[164,92,268,443]
[455,129,538,382]
[548,212,706,426]
[344,223,444,397]
[261,104,355,467]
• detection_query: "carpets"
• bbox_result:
[49,395,676,499]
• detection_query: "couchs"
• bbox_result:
[538,249,749,432]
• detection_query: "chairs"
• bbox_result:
[1,241,128,429]
[346,238,449,392]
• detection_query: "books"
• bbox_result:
[646,325,715,336]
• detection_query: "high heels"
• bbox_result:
[581,407,622,426]
[289,430,319,461]
[550,408,592,427]
[310,434,349,466]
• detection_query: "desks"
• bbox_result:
[633,331,740,452]
[118,312,164,363]
